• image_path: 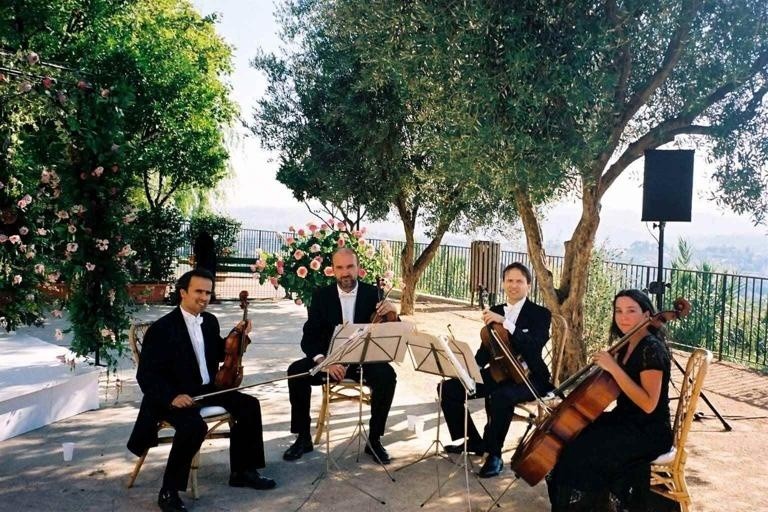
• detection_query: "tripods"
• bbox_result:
[295,381,386,512]
[421,396,501,511]
[311,370,402,485]
[643,282,733,432]
[394,380,471,477]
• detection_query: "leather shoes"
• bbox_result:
[229,469,276,490]
[444,439,485,457]
[479,454,503,478]
[158,486,187,512]
[283,433,314,461]
[365,439,391,464]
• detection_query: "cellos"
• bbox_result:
[511,298,691,486]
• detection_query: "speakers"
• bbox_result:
[642,149,695,222]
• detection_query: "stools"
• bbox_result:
[314,373,372,445]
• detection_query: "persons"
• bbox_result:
[283,247,397,465]
[126,270,276,512]
[547,289,679,512]
[437,261,553,478]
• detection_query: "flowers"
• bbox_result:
[250,218,406,307]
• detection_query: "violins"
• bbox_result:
[214,291,249,389]
[476,284,528,385]
[371,275,397,323]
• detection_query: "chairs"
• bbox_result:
[127,321,234,500]
[483,314,568,460]
[650,348,723,512]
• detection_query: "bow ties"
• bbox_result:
[503,303,521,314]
[339,291,357,297]
[188,316,203,326]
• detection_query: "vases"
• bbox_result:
[307,306,312,320]
